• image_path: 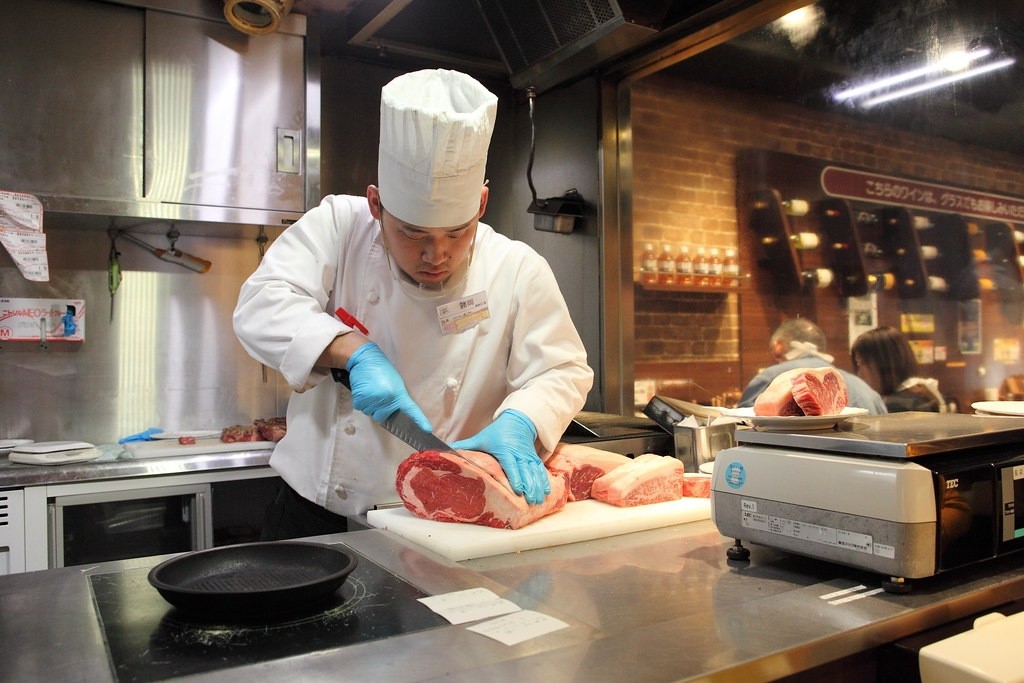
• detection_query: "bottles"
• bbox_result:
[642,243,738,288]
[720,406,869,431]
[751,199,1024,294]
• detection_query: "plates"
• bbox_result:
[971,400,1024,417]
[698,461,715,474]
[0,438,34,456]
[149,430,222,440]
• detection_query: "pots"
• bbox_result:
[147,541,359,616]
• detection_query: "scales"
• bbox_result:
[711,410,1024,595]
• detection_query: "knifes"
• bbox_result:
[330,367,476,468]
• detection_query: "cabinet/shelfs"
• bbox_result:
[0,0,321,239]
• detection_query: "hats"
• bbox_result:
[378,68,498,226]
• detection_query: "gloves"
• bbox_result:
[345,342,433,435]
[448,409,551,506]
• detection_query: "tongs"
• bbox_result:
[367,495,712,561]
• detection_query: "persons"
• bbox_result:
[851,325,947,414]
[232,69,596,547]
[735,318,889,419]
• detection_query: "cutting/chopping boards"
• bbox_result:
[124,438,280,460]
